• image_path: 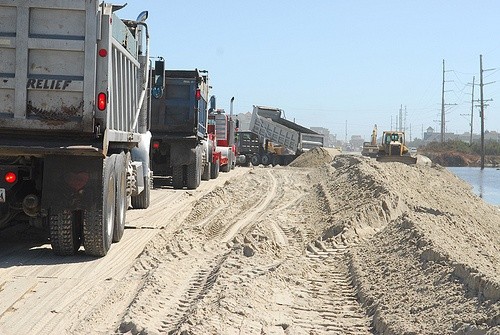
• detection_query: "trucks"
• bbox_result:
[0,0,153,259]
[147,70,219,189]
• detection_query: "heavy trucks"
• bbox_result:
[233,104,325,168]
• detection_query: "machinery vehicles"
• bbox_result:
[215,96,238,172]
[376,131,417,165]
[362,124,380,158]
